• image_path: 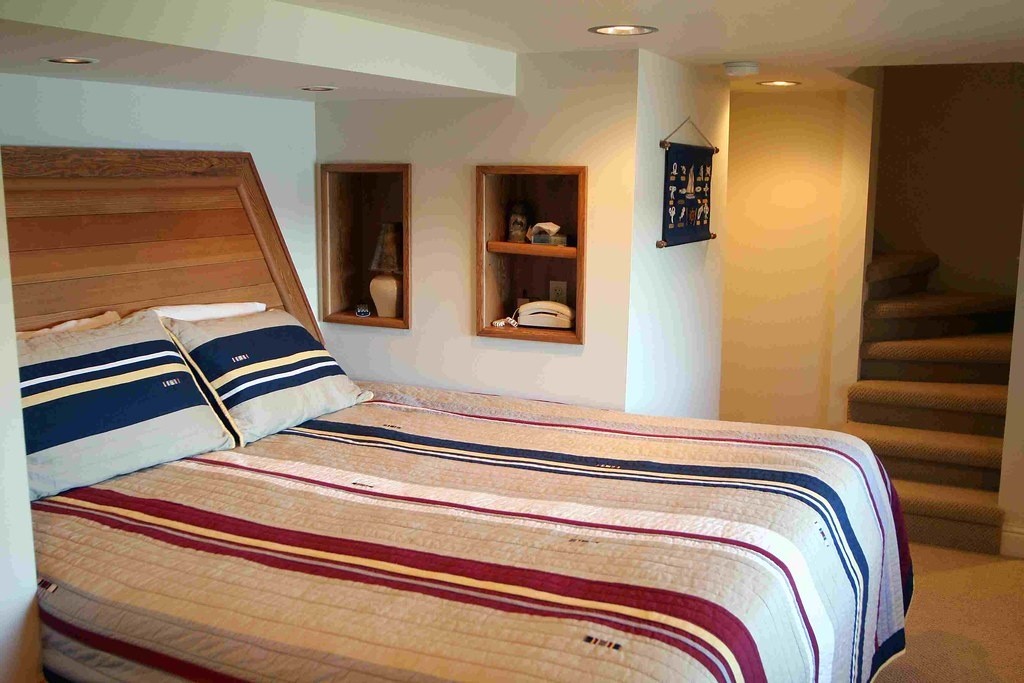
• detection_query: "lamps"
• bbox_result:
[368,219,404,320]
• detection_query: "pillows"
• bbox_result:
[14,308,234,504]
[162,308,375,446]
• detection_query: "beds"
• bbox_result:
[1,137,922,683]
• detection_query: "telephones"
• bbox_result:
[518,300,576,328]
